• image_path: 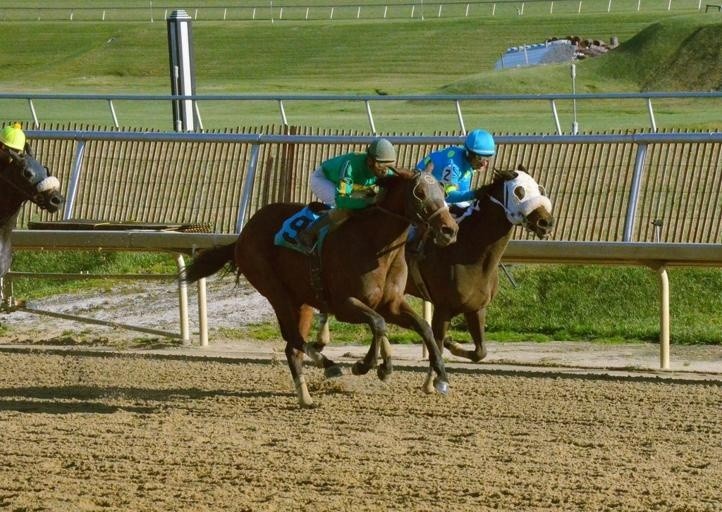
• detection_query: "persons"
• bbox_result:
[297,139,395,247]
[0,125,28,165]
[404,129,494,256]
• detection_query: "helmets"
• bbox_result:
[0,123,25,151]
[368,138,396,162]
[464,129,495,156]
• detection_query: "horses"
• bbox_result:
[0,142,64,276]
[167,159,461,410]
[311,164,555,395]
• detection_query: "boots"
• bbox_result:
[300,212,330,247]
